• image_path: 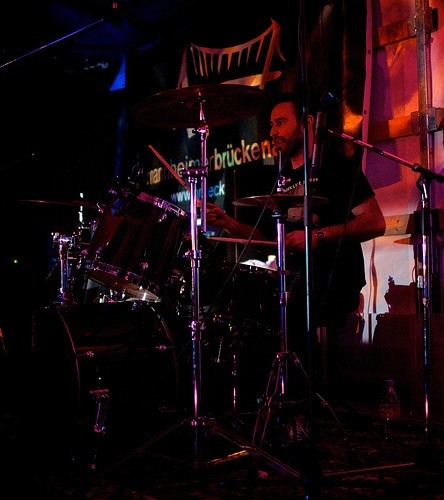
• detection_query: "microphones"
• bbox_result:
[308,110,327,178]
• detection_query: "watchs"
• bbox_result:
[315,228,326,244]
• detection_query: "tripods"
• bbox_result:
[27,153,443,490]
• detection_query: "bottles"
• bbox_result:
[378,379,400,444]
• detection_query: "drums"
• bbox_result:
[231,271,299,332]
[86,189,191,301]
[30,301,180,412]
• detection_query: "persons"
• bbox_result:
[201,92,386,417]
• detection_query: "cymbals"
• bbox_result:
[233,194,329,209]
[130,82,274,129]
[19,197,100,210]
